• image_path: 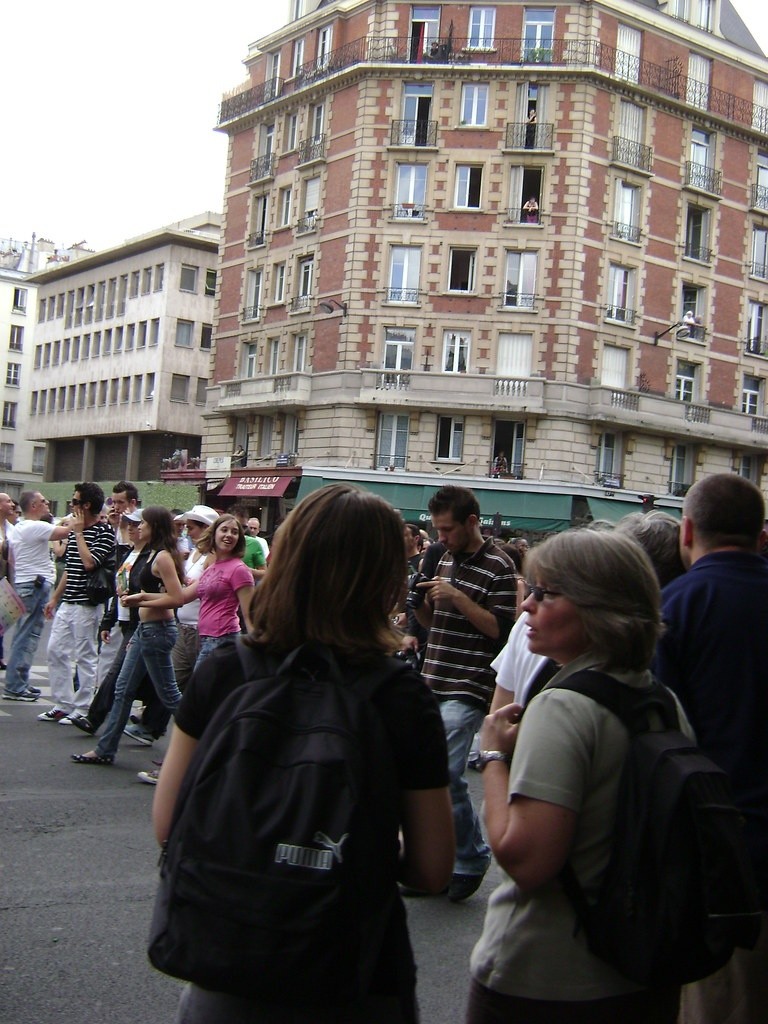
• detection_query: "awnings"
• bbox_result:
[218,476,295,497]
[293,475,682,533]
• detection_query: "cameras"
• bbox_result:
[404,571,436,609]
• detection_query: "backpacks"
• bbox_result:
[146,634,414,1004]
[543,668,760,987]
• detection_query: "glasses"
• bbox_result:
[71,498,80,506]
[522,580,562,602]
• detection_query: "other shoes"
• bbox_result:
[153,760,163,766]
[72,717,94,734]
[71,753,113,765]
[0,661,7,669]
[131,714,141,724]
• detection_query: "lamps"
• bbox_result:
[655,321,690,344]
[318,299,347,316]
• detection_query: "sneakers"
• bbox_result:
[58,711,88,725]
[28,686,41,694]
[137,770,160,785]
[37,710,69,721]
[123,726,154,747]
[448,873,483,901]
[3,689,40,702]
[397,880,448,897]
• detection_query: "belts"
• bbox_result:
[68,601,91,606]
[142,621,177,629]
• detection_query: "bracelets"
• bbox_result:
[76,533,83,537]
[467,751,509,772]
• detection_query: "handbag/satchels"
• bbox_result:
[86,521,119,605]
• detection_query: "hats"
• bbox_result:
[173,505,219,527]
[124,508,144,521]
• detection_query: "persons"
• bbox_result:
[151,483,456,1024]
[620,473,768,1024]
[234,445,246,467]
[468,529,698,1024]
[523,196,538,222]
[527,109,536,124]
[495,450,507,472]
[0,479,269,785]
[683,311,702,337]
[390,484,552,904]
